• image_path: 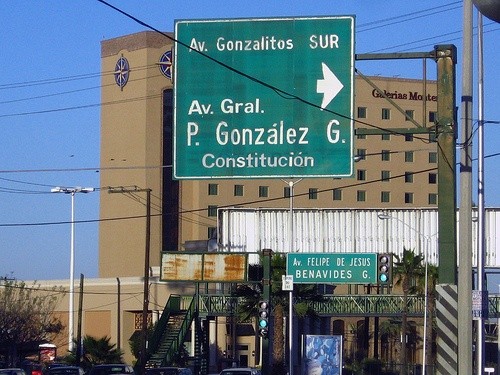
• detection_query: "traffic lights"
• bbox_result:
[378,254,393,288]
[258,300,270,337]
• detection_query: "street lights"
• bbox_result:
[51,186,93,355]
[378,215,478,375]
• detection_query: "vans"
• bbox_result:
[47,367,83,375]
[85,364,133,375]
[145,367,192,375]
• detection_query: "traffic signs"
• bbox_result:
[172,16,355,180]
[287,253,378,284]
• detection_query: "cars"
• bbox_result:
[219,368,260,375]
